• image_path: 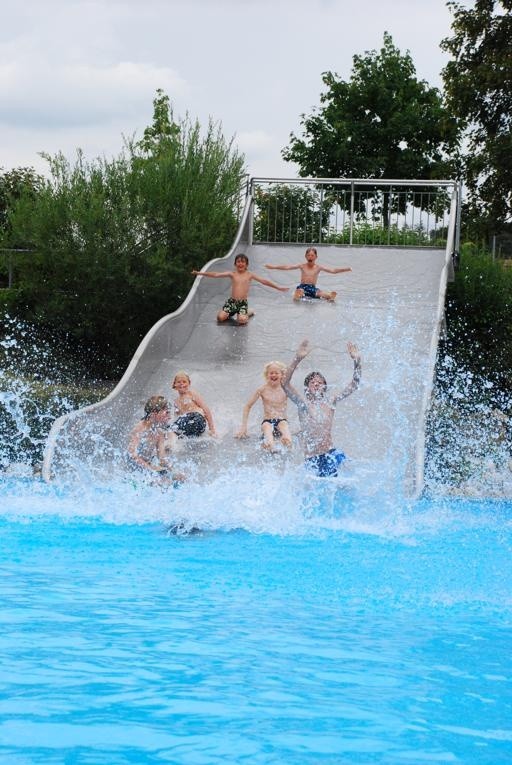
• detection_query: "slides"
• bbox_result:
[42,238,457,497]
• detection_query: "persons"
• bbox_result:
[232,360,306,454]
[160,374,218,451]
[280,339,362,479]
[189,253,291,324]
[264,247,352,301]
[114,395,188,493]
